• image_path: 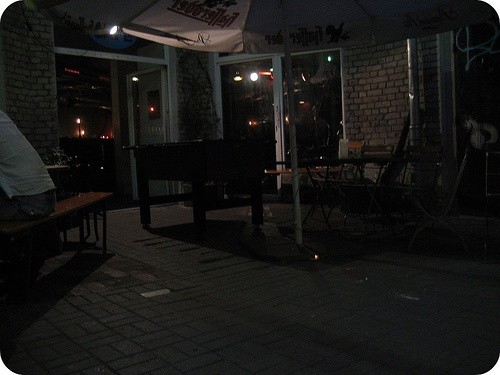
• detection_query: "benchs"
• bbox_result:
[0,191,113,306]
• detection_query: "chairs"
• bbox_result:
[263,144,500,264]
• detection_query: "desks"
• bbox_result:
[46,164,71,199]
[120,137,278,240]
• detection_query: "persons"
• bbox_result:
[0,110,56,302]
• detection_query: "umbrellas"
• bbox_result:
[51,0,498,262]
